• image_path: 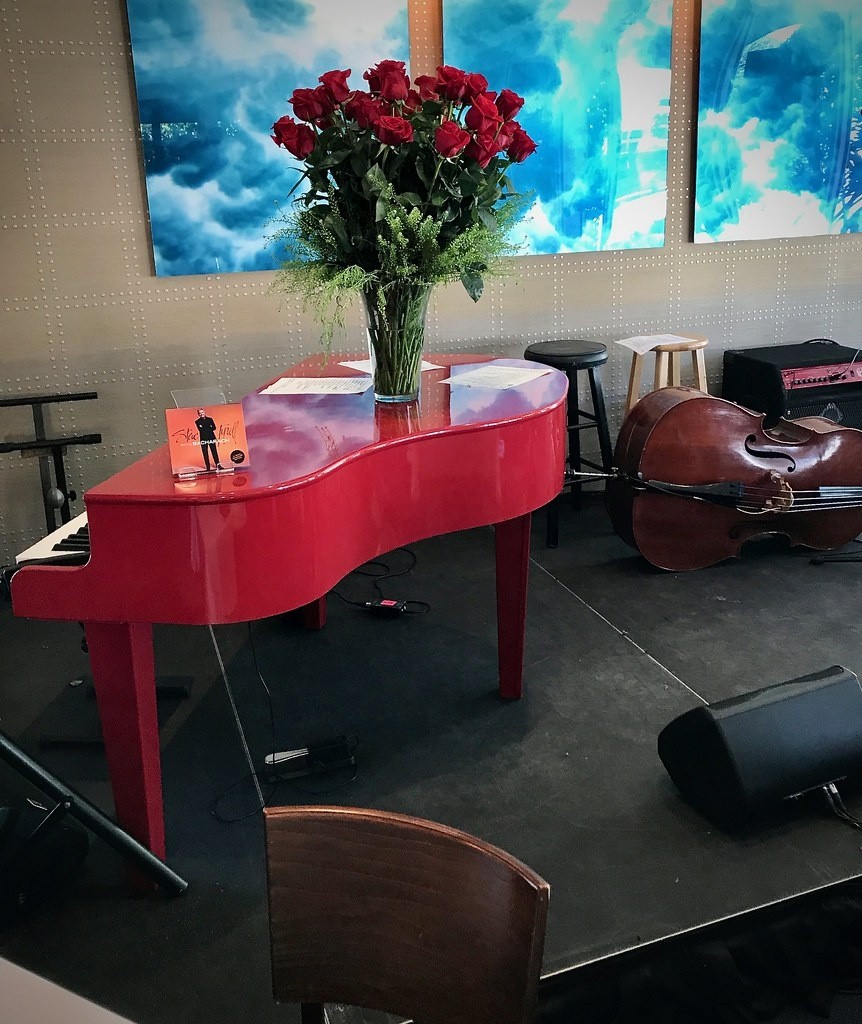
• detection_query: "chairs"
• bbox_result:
[263,804,556,1024]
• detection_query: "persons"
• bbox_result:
[194,407,225,471]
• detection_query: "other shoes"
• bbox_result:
[216,464,224,469]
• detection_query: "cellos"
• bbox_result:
[570,383,861,573]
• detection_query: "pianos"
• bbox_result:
[8,349,571,884]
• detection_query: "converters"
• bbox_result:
[362,599,408,615]
[264,736,354,783]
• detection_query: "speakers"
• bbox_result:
[656,664,862,823]
[722,337,862,428]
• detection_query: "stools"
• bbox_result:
[522,340,615,552]
[623,331,710,421]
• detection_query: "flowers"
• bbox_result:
[267,60,539,269]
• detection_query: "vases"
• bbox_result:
[354,275,436,404]
